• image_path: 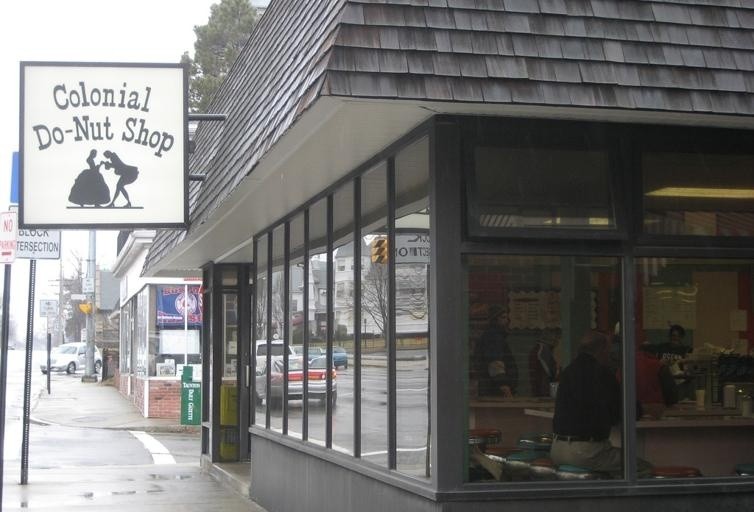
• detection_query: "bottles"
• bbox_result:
[722,383,753,418]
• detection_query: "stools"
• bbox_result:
[468,427,754,478]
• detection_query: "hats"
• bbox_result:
[487,360,507,378]
[578,331,609,356]
[487,303,507,320]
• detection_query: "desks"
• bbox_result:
[524,403,754,475]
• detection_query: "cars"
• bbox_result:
[7,342,16,350]
[256,333,346,408]
[40,341,103,375]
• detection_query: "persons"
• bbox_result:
[616,344,667,415]
[656,325,690,358]
[549,331,654,480]
[478,305,518,399]
[529,327,562,397]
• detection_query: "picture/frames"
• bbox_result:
[504,286,600,334]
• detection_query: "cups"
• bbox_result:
[695,385,706,407]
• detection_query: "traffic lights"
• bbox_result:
[371,238,385,263]
[78,303,92,314]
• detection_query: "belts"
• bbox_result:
[553,434,581,441]
